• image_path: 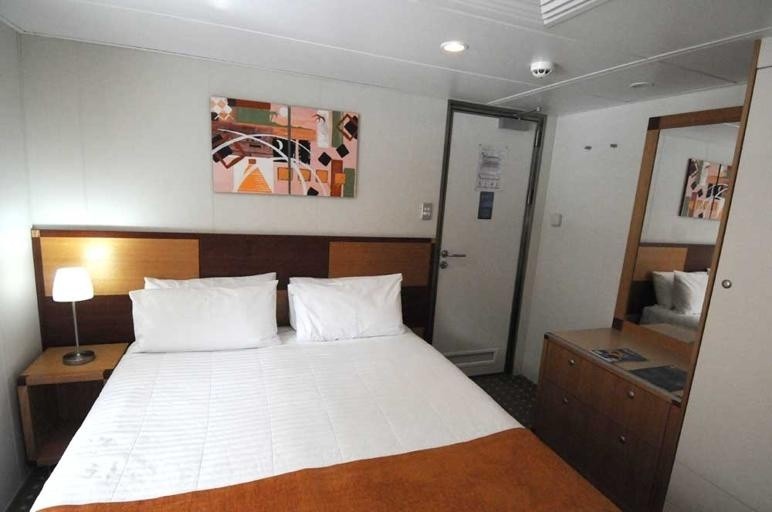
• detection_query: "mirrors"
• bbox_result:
[609,105,746,368]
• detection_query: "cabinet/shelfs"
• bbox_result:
[533,328,695,511]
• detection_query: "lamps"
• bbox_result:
[53,261,95,368]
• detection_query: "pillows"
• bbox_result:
[647,266,707,313]
[128,261,410,356]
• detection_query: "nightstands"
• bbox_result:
[12,340,130,465]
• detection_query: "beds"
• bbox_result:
[25,227,631,512]
